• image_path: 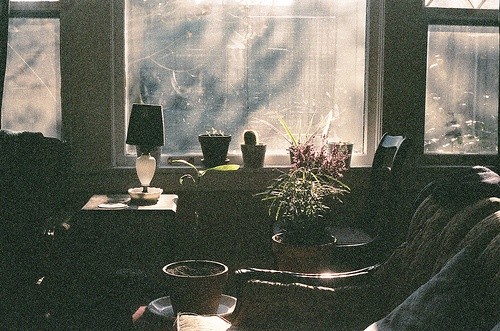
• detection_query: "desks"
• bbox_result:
[80,193,180,261]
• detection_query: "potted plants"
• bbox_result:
[161,159,240,316]
[241,129,268,168]
[198,127,232,167]
[253,107,333,163]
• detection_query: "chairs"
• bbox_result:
[273,132,413,269]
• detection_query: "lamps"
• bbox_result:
[125,103,166,200]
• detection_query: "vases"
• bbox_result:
[328,143,353,166]
[271,232,337,273]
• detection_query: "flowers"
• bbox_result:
[256,133,350,243]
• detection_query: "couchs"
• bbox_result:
[233,166,499,330]
